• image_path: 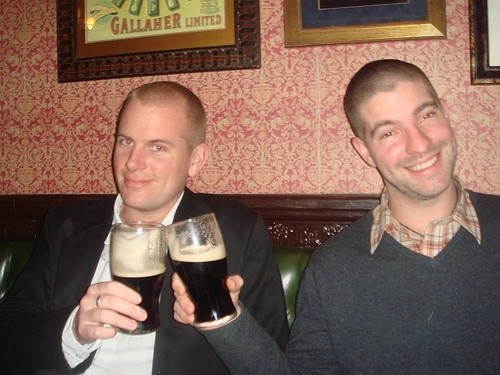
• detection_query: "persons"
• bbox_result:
[172,59,500,375]
[0,82,289,375]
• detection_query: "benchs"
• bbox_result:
[0,193,380,331]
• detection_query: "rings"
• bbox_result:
[97,295,101,308]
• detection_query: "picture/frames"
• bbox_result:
[468,0,500,85]
[56,0,261,83]
[283,0,447,48]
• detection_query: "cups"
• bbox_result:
[110,221,167,335]
[165,212,237,329]
